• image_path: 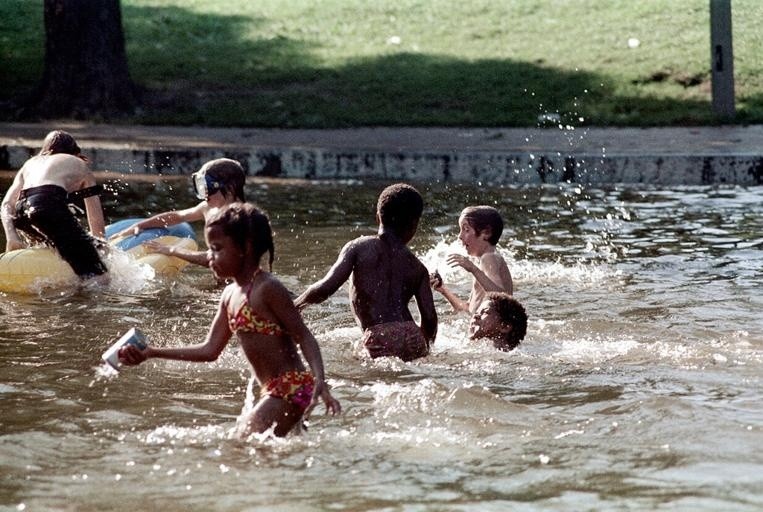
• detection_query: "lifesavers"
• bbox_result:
[0,219,197,293]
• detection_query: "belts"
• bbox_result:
[19,184,69,199]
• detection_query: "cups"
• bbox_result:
[101,327,148,373]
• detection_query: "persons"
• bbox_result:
[0,129,106,288]
[465,291,527,352]
[293,184,437,366]
[107,158,247,288]
[430,204,513,320]
[102,203,341,457]
